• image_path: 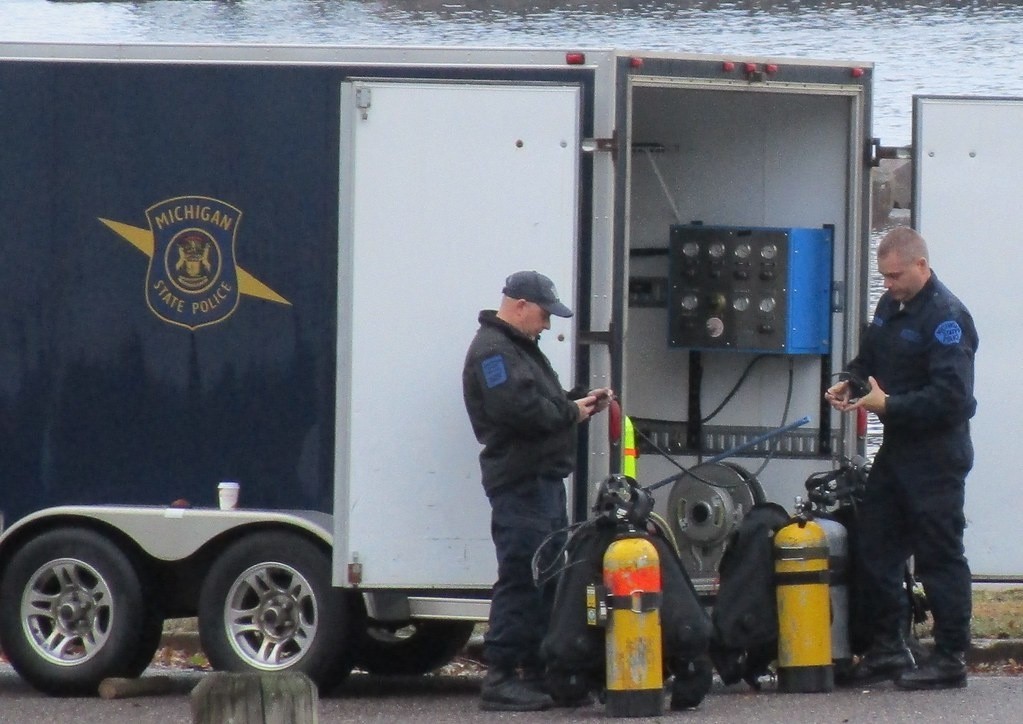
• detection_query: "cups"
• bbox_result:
[217,482,239,510]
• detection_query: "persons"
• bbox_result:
[463,271,615,711]
[824,227,979,689]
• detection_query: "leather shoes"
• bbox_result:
[895,649,966,690]
[478,666,553,712]
[834,648,916,686]
[515,665,590,706]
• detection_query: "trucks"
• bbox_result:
[1,33,1023,698]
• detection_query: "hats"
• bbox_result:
[502,270,574,318]
